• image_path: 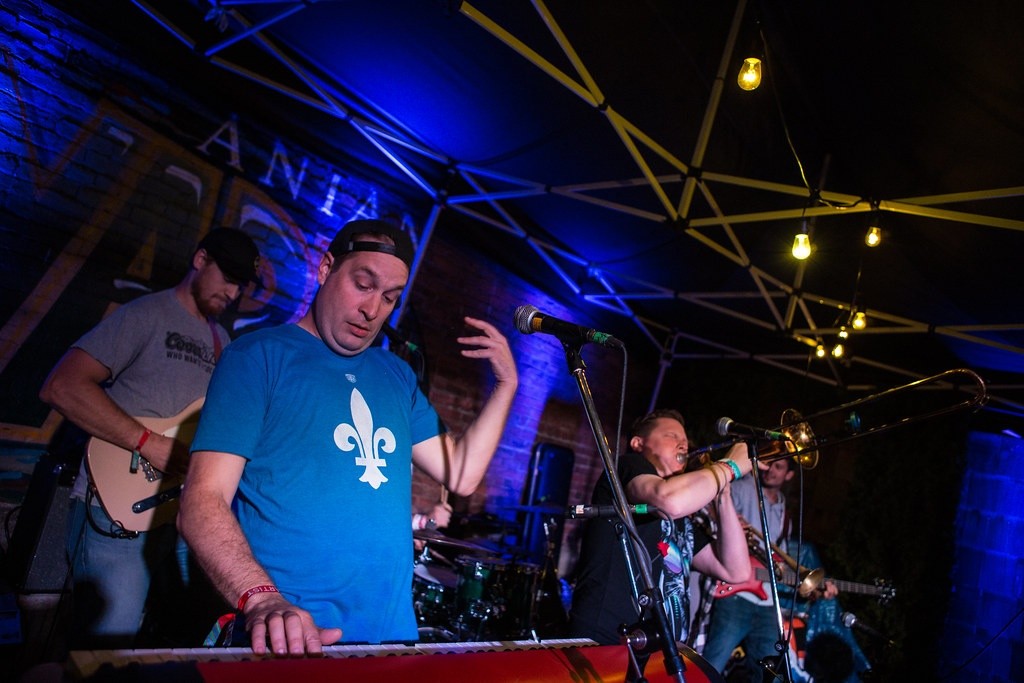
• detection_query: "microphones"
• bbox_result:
[716,416,791,441]
[570,502,659,519]
[842,611,895,645]
[514,304,625,349]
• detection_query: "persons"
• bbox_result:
[41,224,261,645]
[699,450,838,676]
[569,408,769,642]
[410,499,453,558]
[176,218,519,660]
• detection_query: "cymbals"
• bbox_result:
[412,529,496,554]
[496,502,569,515]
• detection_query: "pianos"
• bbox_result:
[63,634,721,683]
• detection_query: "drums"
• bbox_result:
[488,561,541,637]
[417,623,471,643]
[409,561,465,624]
[456,553,511,621]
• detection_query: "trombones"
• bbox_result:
[674,366,991,471]
[738,517,825,595]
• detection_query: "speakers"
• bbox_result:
[6,463,89,594]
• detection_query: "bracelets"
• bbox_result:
[704,461,727,494]
[133,429,151,452]
[718,457,741,482]
[236,586,280,612]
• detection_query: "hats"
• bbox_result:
[200,226,268,290]
[328,220,414,288]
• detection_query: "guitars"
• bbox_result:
[713,552,898,606]
[84,394,209,534]
